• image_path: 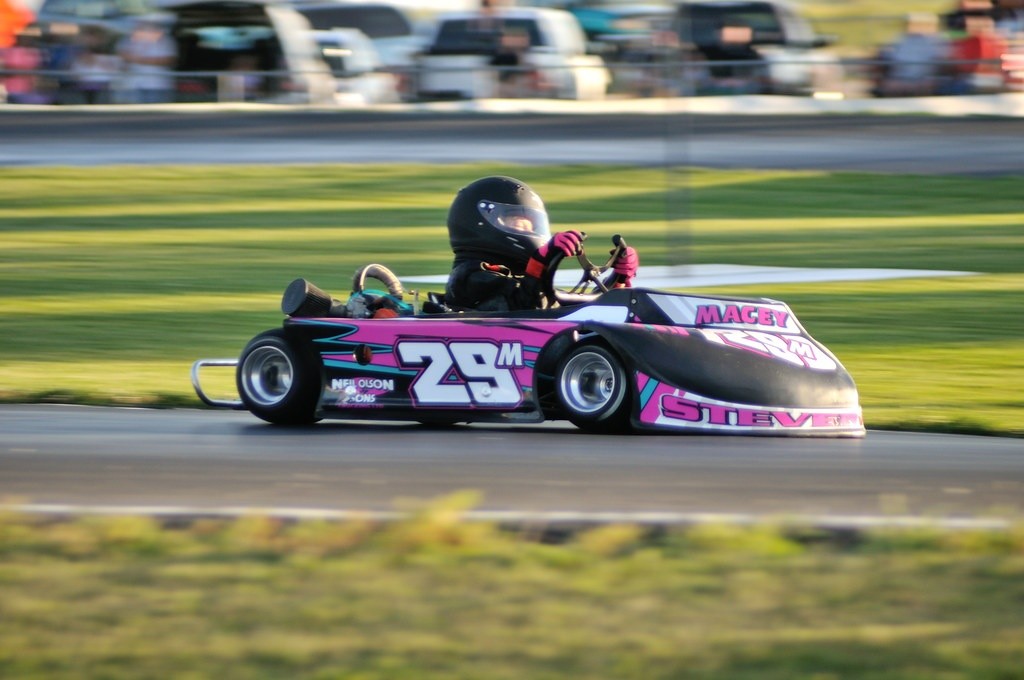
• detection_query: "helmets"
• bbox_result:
[448,176,552,254]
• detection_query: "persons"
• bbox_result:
[54,21,178,104]
[444,175,639,311]
[877,0,1024,96]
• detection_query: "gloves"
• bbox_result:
[531,230,585,266]
[609,247,640,287]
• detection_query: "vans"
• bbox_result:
[666,1,838,102]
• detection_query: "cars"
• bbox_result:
[171,5,433,106]
[404,7,613,100]
